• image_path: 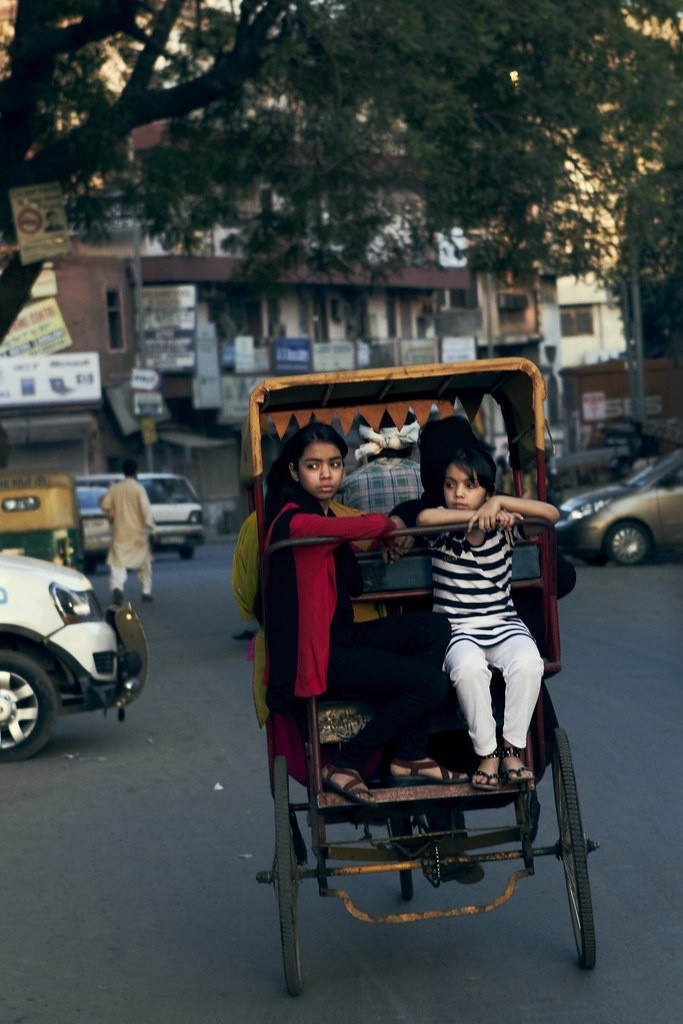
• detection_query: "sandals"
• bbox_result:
[500,750,535,784]
[387,761,468,782]
[472,752,500,789]
[321,764,376,804]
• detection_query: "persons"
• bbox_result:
[334,410,433,594]
[265,420,469,807]
[388,415,480,546]
[417,447,560,790]
[627,420,648,467]
[99,459,159,604]
[232,455,388,729]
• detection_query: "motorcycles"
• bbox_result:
[0,470,86,572]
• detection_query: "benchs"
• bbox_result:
[277,533,555,759]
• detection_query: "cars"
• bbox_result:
[77,484,113,570]
[555,443,683,567]
[0,549,149,764]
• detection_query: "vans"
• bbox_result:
[76,474,205,559]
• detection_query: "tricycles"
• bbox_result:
[247,358,598,994]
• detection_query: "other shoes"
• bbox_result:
[113,589,124,607]
[141,595,155,603]
